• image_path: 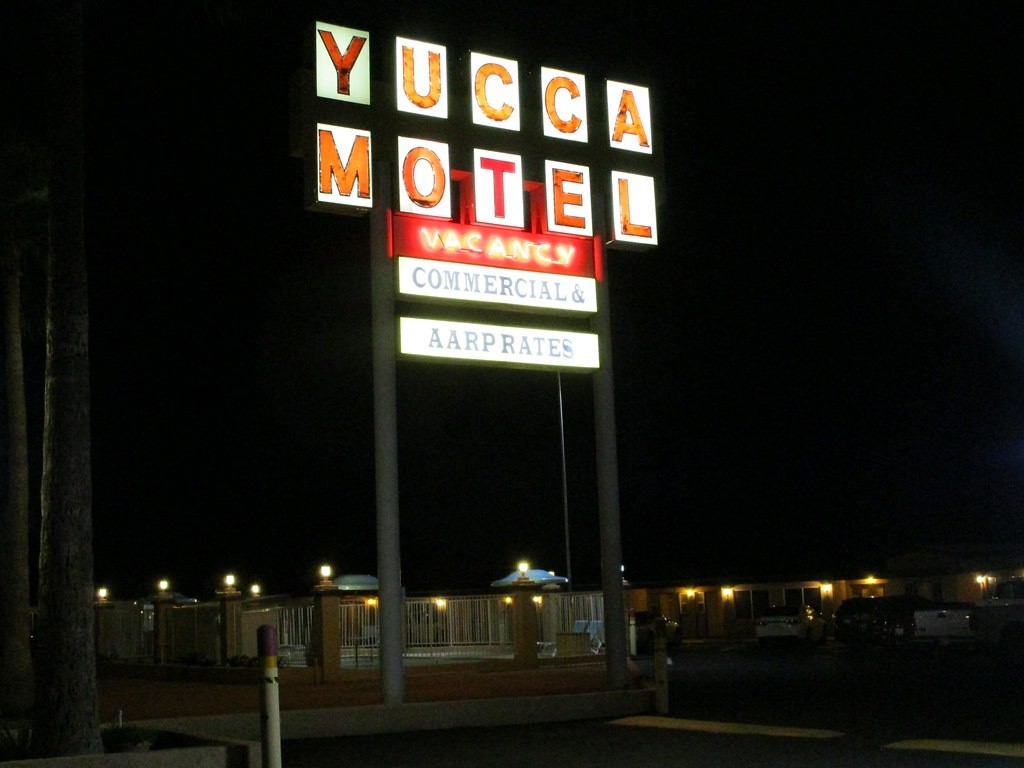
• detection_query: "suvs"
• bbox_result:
[829,595,931,648]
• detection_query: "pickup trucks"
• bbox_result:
[911,600,976,649]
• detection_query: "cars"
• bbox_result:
[635,611,682,648]
[754,605,828,647]
[971,575,1024,648]
